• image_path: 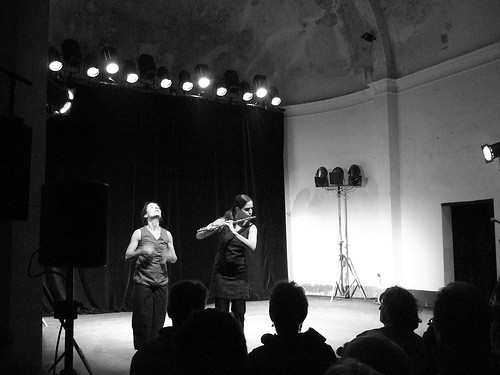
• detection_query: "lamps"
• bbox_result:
[481,142,500,163]
[314,165,362,195]
[49,39,281,113]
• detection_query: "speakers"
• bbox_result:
[39,180,108,267]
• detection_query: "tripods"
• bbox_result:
[325,185,366,301]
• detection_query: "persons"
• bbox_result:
[196,194,258,336]
[125,201,177,351]
[327,333,409,375]
[245,281,337,375]
[421,281,500,375]
[130,280,249,375]
[336,284,423,362]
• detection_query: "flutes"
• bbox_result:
[196,215,256,231]
